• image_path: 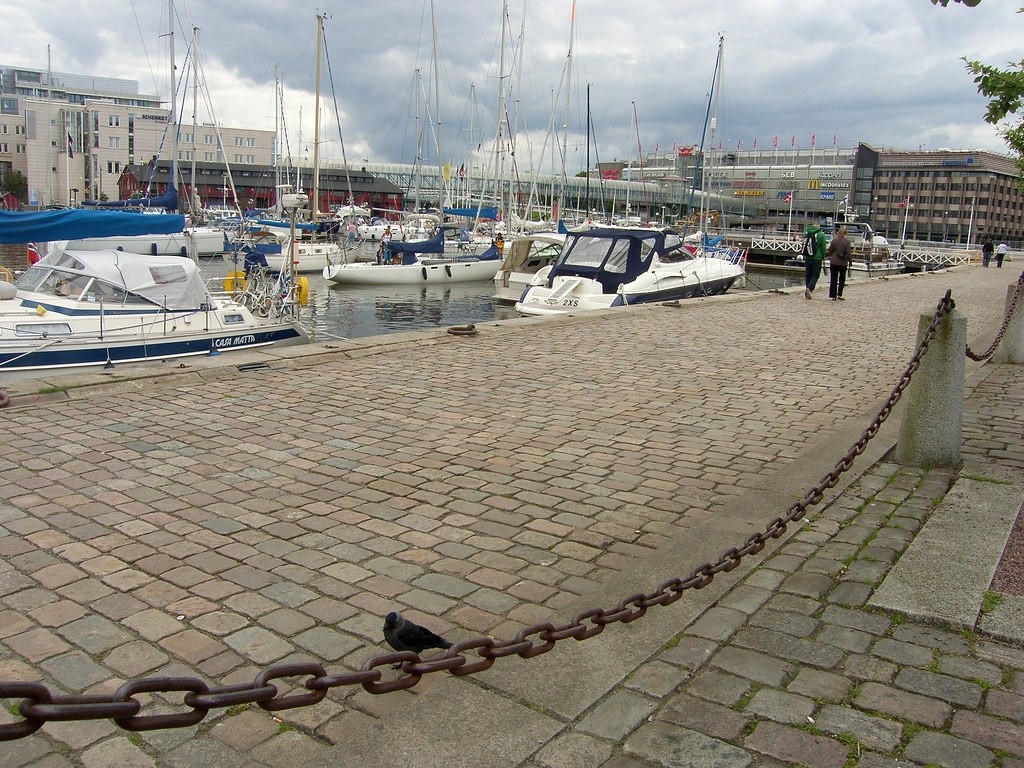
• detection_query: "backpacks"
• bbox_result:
[800,230,820,257]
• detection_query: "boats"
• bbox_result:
[816,223,909,285]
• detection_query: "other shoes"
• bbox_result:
[831,297,845,301]
[804,288,812,300]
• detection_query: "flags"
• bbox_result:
[455,167,459,176]
[443,162,450,182]
[839,196,848,204]
[637,133,837,151]
[29,242,44,264]
[68,134,75,160]
[783,193,791,203]
[464,165,468,177]
[897,198,908,208]
[459,163,464,182]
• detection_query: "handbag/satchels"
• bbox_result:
[837,250,850,260]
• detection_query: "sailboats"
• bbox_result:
[0,2,751,376]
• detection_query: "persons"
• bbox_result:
[982,240,994,269]
[552,198,558,222]
[339,191,543,287]
[224,200,257,221]
[994,241,1012,268]
[801,220,828,300]
[828,227,853,302]
[299,208,342,243]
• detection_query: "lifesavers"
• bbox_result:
[297,277,308,306]
[224,271,246,298]
